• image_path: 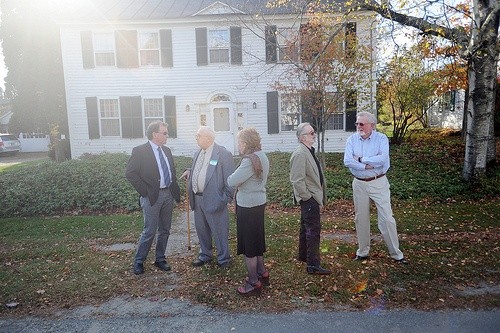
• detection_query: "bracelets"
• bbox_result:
[358,156,362,163]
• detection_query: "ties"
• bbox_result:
[192,151,206,194]
[157,147,170,186]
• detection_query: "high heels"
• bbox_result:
[245,271,270,285]
[237,280,261,297]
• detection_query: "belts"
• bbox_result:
[160,186,170,190]
[355,173,385,181]
[193,192,203,196]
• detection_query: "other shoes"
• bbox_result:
[392,258,408,265]
[354,255,369,261]
[155,260,171,271]
[307,265,332,274]
[133,262,144,274]
[193,259,204,266]
[219,262,228,269]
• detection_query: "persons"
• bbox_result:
[227,128,269,296]
[344,112,412,266]
[179,126,236,268]
[290,123,332,275]
[125,121,180,276]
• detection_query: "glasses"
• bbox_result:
[302,131,316,135]
[355,122,372,126]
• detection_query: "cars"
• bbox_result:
[0,133,22,156]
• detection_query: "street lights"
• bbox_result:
[370,17,382,114]
